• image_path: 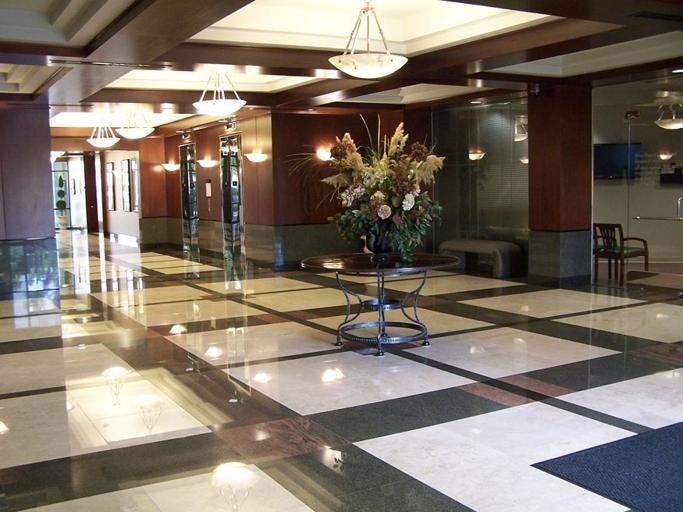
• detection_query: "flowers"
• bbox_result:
[282,114,446,262]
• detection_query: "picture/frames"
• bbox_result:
[121,159,131,212]
[105,162,116,211]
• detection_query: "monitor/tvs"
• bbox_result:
[594,142,642,180]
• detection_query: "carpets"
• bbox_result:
[530,421,683,511]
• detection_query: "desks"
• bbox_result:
[300,252,463,356]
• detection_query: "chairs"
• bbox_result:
[593,224,648,286]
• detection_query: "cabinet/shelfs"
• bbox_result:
[658,163,683,184]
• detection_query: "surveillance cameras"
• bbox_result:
[530,83,540,96]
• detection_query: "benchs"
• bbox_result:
[439,240,522,279]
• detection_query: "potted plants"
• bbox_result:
[56,175,68,225]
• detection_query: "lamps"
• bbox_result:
[192,64,247,116]
[86,111,120,148]
[160,157,181,171]
[653,104,683,130]
[468,146,486,160]
[514,119,528,142]
[115,103,155,140]
[519,153,529,164]
[328,0,409,79]
[196,153,220,168]
[244,148,272,163]
